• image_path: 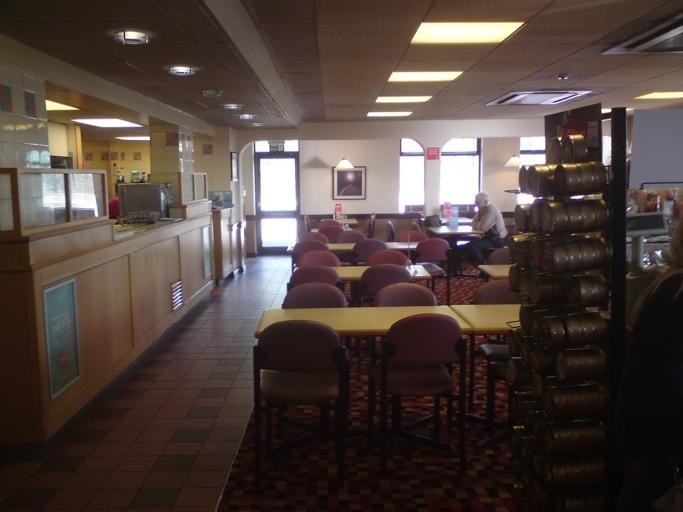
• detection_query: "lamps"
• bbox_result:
[335,159,354,170]
[506,137,524,168]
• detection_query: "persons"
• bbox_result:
[611,215,683,512]
[465,193,508,278]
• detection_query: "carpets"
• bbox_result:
[213,258,532,512]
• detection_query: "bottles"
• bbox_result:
[140,172,146,183]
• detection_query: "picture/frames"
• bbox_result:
[333,167,367,200]
[232,152,239,181]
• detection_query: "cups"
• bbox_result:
[510,135,614,512]
